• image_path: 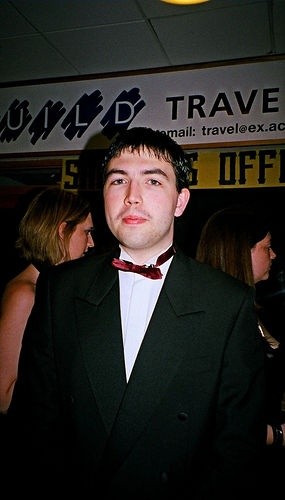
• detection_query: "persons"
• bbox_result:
[0,189,95,415]
[0,127,267,500]
[194,207,285,445]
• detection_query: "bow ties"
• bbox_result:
[112,246,176,280]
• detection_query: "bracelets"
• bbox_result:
[272,424,284,444]
